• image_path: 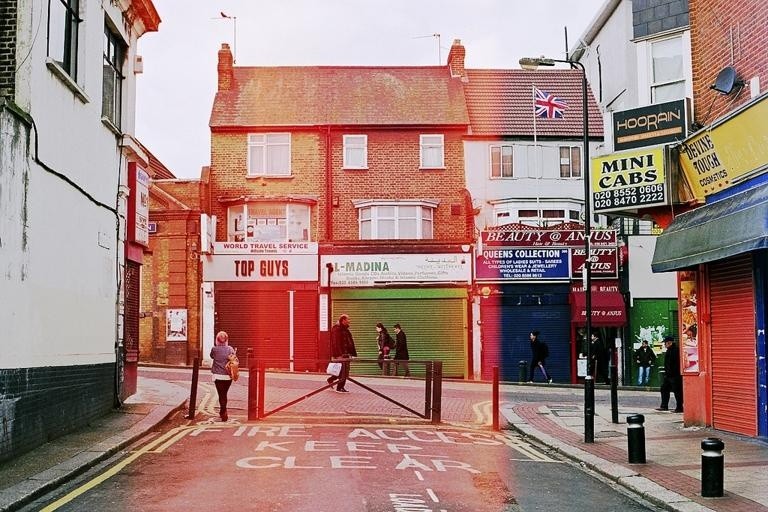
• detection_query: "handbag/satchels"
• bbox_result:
[385,335,395,349]
[326,361,342,377]
[226,352,241,382]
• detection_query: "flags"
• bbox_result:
[535,88,568,120]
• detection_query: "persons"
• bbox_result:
[374,323,394,374]
[635,341,656,384]
[328,314,357,394]
[654,335,685,413]
[526,332,554,383]
[208,330,240,423]
[587,333,608,383]
[389,327,413,378]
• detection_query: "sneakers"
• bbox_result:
[336,387,349,393]
[327,378,334,389]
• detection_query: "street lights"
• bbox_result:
[325,260,334,371]
[518,54,597,444]
